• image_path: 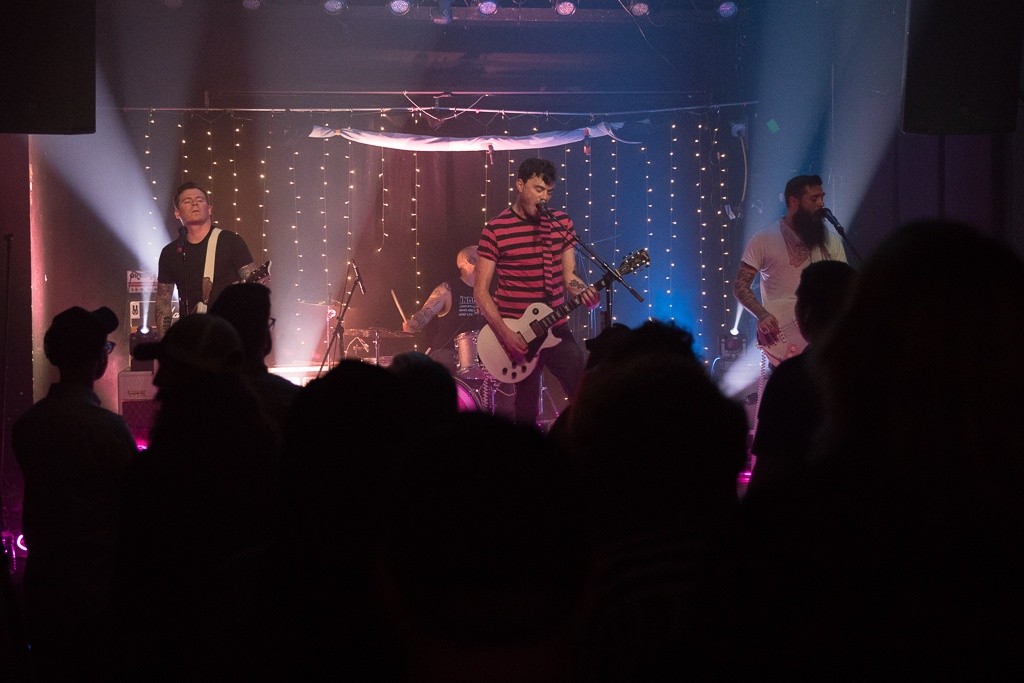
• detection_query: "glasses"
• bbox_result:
[465,256,476,266]
[266,317,276,329]
[101,341,115,353]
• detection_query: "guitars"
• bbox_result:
[241,259,273,284]
[476,247,650,384]
[757,297,808,360]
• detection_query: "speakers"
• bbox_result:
[899,0,1024,135]
[0,0,97,133]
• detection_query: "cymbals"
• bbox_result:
[347,328,415,339]
[297,298,359,310]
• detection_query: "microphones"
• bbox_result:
[176,226,188,254]
[820,207,844,236]
[535,200,548,216]
[352,263,366,294]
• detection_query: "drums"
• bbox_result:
[430,331,487,379]
[452,376,481,414]
[364,356,394,369]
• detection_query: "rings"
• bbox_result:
[763,330,768,335]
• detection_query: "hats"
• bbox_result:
[44,306,119,360]
[585,323,630,349]
[134,315,245,373]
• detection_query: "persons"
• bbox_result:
[402,245,497,377]
[732,174,850,372]
[473,157,601,422]
[0,217,1024,683]
[154,181,258,340]
